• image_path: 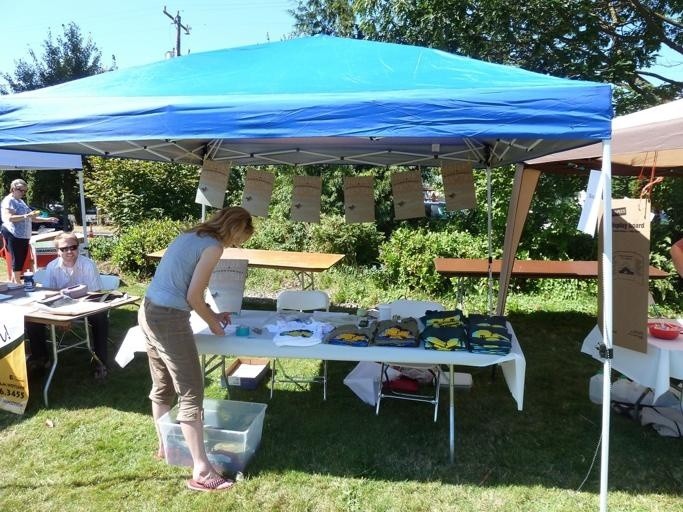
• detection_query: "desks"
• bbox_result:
[116,310,526,464]
[147,248,346,291]
[581,319,683,438]
[433,258,669,315]
[0,284,141,410]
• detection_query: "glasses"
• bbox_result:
[17,189,27,193]
[58,245,79,252]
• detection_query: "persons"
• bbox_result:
[671,239,683,277]
[28,233,108,377]
[1,179,59,283]
[139,206,255,492]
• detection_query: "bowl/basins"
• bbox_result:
[648,323,682,340]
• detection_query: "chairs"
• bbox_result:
[376,301,444,423]
[91,275,120,366]
[202,261,248,401]
[271,291,329,400]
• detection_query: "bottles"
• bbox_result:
[24,269,35,291]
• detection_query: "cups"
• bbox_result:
[236,327,249,336]
[379,305,391,320]
[356,309,367,317]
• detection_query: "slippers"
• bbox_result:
[187,476,234,492]
[95,369,107,376]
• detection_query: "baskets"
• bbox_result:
[647,321,682,339]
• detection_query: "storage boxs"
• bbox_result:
[220,359,270,390]
[156,399,268,472]
